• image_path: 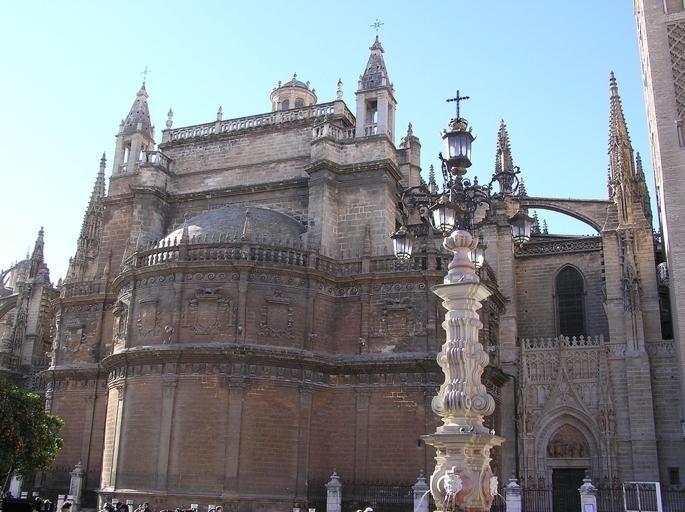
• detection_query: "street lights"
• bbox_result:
[393,118,534,511]
